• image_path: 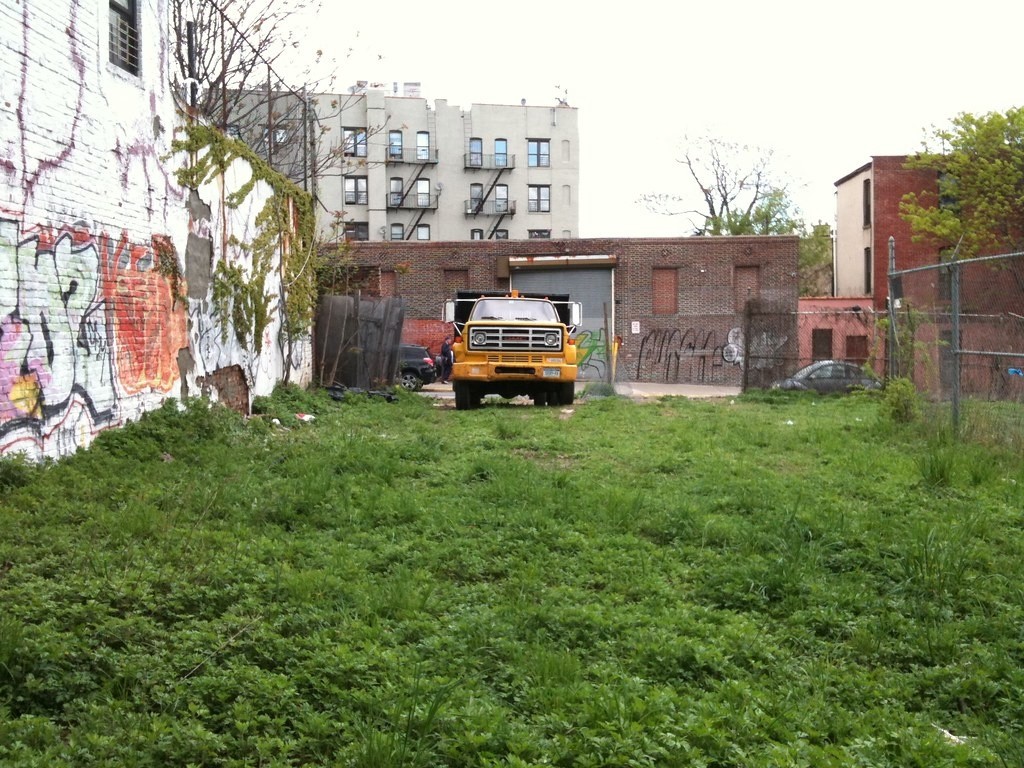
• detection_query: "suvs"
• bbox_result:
[395,343,438,392]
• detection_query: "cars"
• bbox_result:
[770,356,884,396]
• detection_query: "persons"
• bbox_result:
[440,336,453,384]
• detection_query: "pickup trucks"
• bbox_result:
[440,288,583,411]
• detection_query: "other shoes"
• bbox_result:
[442,381,448,384]
